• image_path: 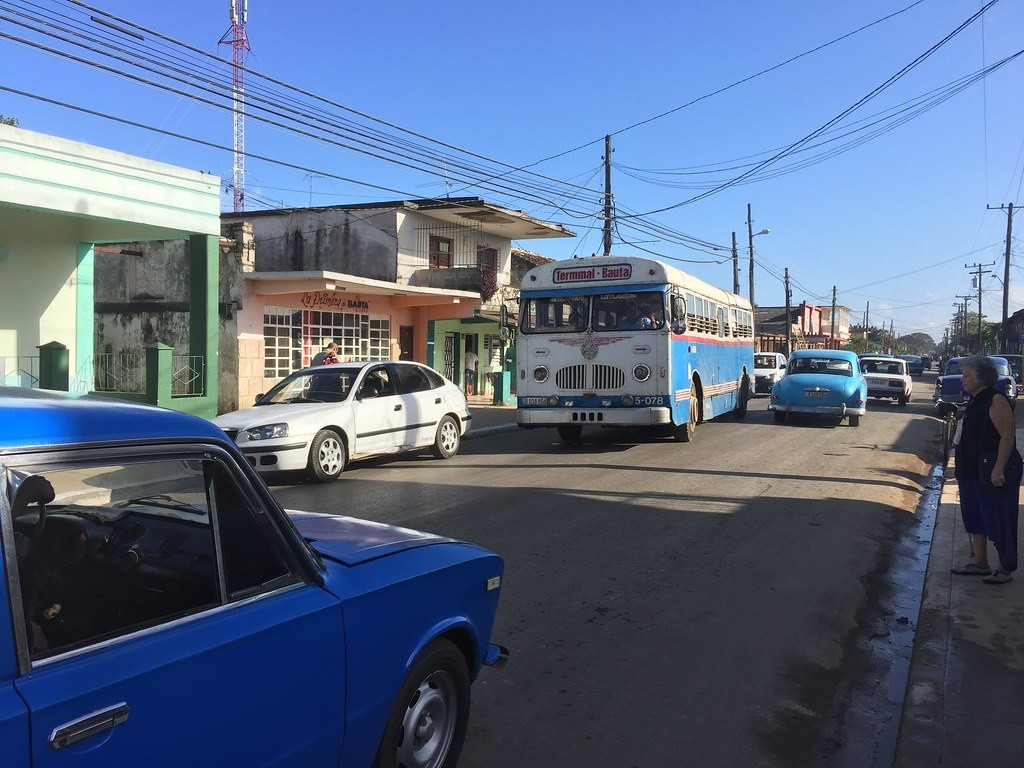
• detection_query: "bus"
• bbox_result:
[500,254,755,443]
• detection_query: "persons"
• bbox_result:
[954,355,1024,584]
[793,358,813,371]
[618,305,657,330]
[311,342,340,370]
[10,476,55,655]
[465,347,479,396]
[769,359,775,368]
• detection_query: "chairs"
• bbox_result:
[307,376,341,398]
[362,377,385,394]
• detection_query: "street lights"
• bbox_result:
[748,229,769,314]
[992,274,1009,355]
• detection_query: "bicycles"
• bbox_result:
[934,398,968,460]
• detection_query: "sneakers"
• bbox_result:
[950,563,991,575]
[982,570,1013,583]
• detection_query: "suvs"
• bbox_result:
[987,355,1022,382]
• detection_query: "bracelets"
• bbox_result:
[651,321,655,323]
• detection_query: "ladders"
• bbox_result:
[792,327,809,350]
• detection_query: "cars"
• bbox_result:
[920,357,931,370]
[936,357,1018,409]
[767,349,867,427]
[754,352,787,397]
[898,355,923,375]
[859,357,913,407]
[0,384,513,768]
[206,361,472,484]
